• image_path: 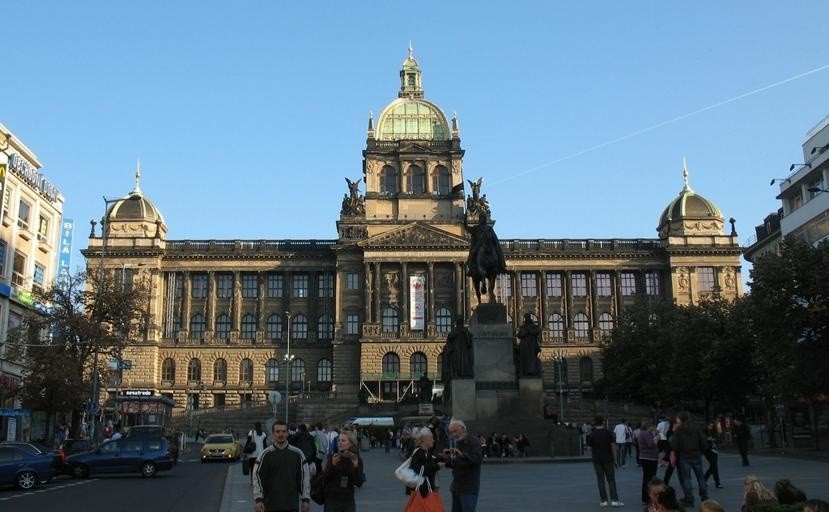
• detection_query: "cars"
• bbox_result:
[0,424,181,492]
[200,433,241,463]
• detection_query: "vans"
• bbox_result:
[344,416,395,432]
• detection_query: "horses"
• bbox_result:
[469,225,502,304]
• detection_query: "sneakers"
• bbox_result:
[701,482,709,487]
[611,501,624,507]
[714,485,724,488]
[678,498,695,508]
[600,501,608,507]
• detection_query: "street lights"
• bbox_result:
[775,400,786,449]
[184,386,193,438]
[806,187,829,194]
[553,355,565,425]
[282,310,296,424]
[88,192,145,441]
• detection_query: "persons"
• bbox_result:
[515,311,540,377]
[343,177,366,212]
[557,413,828,512]
[464,179,490,213]
[442,316,472,378]
[244,408,528,511]
[462,209,508,276]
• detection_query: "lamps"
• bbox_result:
[790,163,811,171]
[811,146,829,154]
[770,177,791,185]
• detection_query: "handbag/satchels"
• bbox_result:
[395,455,426,488]
[311,472,327,505]
[242,441,255,474]
[404,489,445,512]
